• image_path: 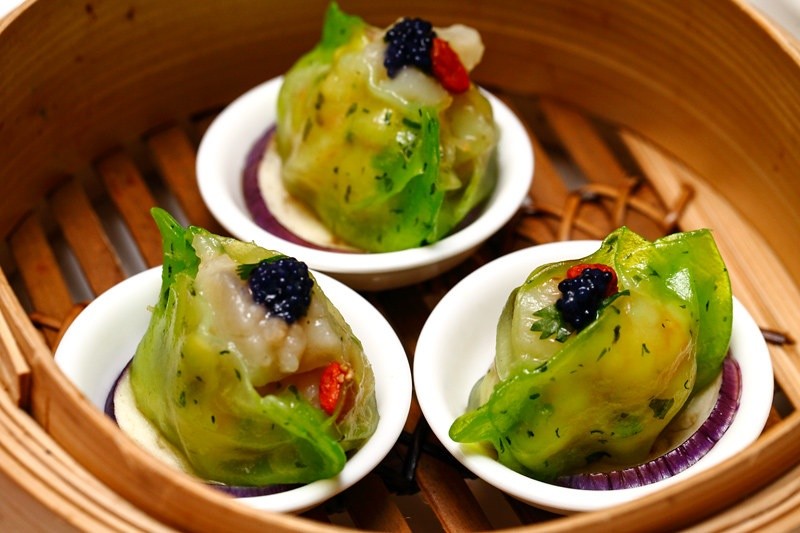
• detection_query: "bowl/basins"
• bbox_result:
[413,241,775,513]
[196,75,533,294]
[54,265,413,516]
